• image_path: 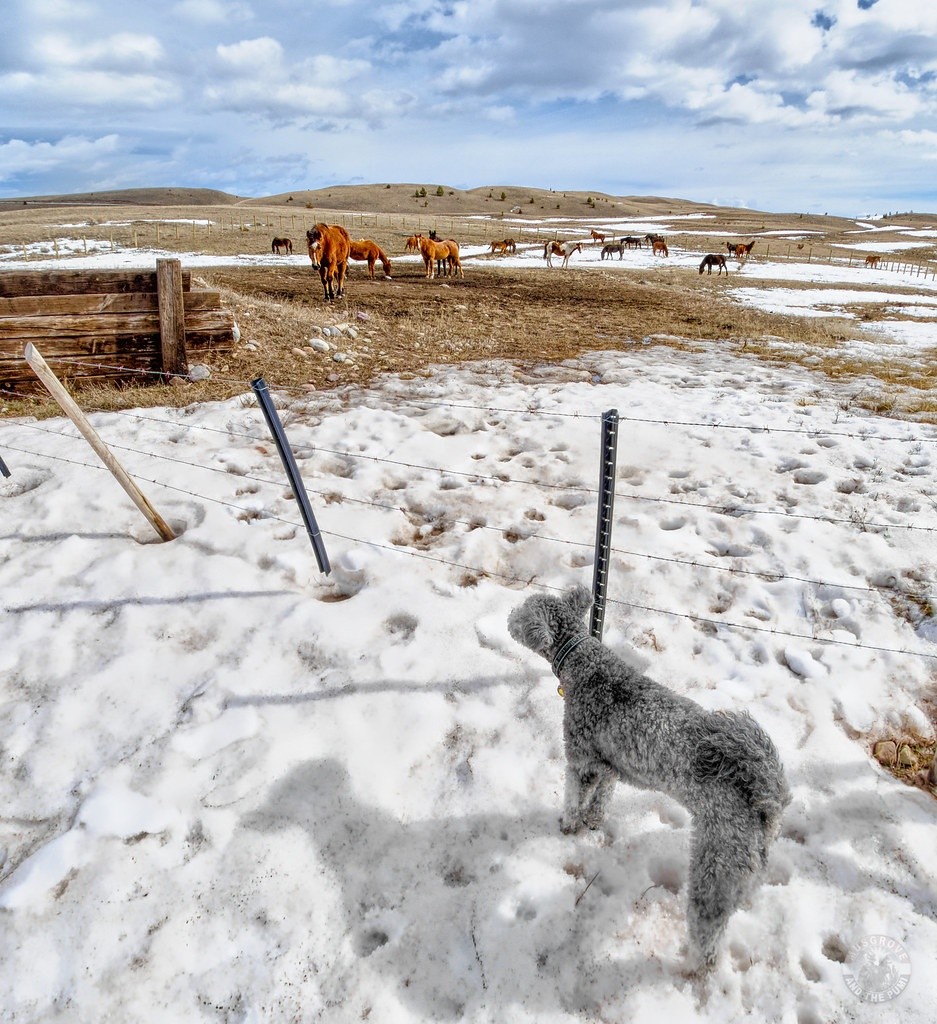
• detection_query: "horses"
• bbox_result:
[698,254,728,277]
[487,238,516,253]
[865,256,882,268]
[645,233,668,257]
[620,237,642,250]
[306,222,391,300]
[545,241,582,269]
[591,230,606,244]
[271,236,292,254]
[726,240,758,258]
[601,244,625,260]
[402,229,466,279]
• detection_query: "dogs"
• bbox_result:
[505,585,790,983]
